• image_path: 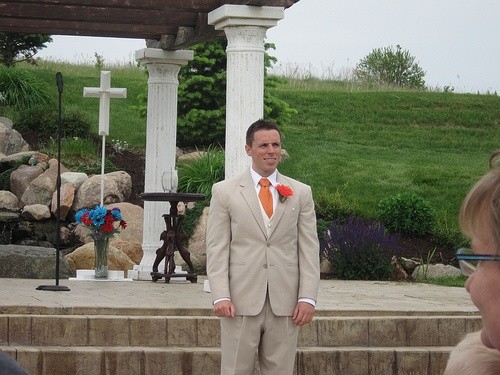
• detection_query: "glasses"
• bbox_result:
[455,247,500,277]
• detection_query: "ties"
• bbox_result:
[258,178,273,219]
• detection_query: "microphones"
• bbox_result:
[56,72,63,92]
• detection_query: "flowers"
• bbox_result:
[74,206,128,241]
[275,183,294,203]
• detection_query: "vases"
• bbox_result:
[92,236,109,278]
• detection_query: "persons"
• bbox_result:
[205,118,320,375]
[442,150,500,375]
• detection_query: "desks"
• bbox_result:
[140,193,205,284]
